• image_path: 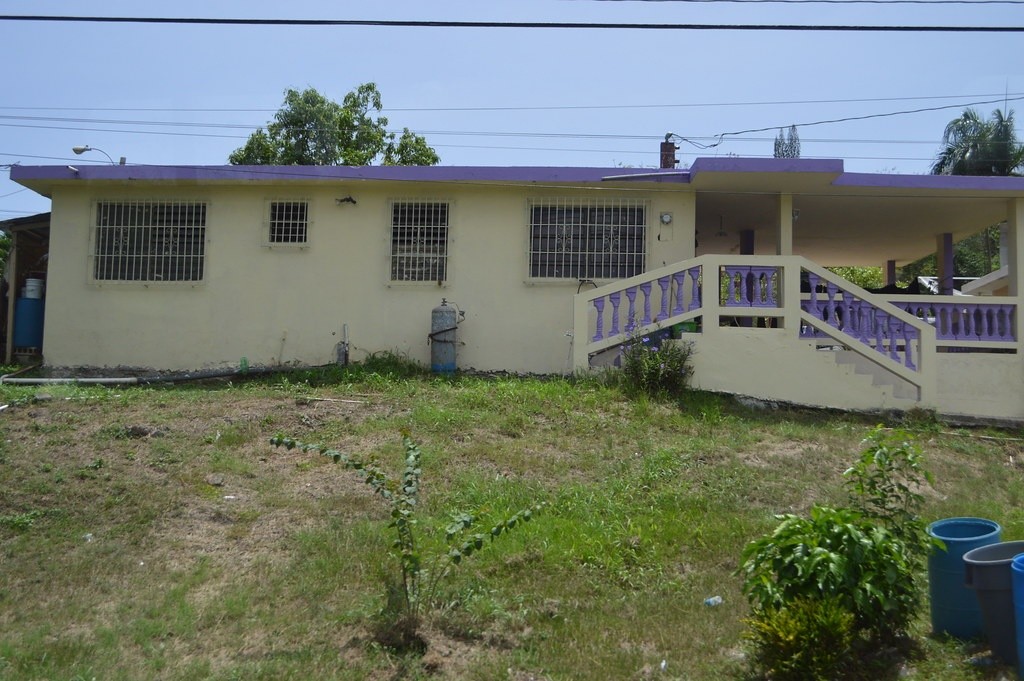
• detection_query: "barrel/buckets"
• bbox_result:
[962,540,1024,665]
[1010,552,1024,676]
[925,516,1001,640]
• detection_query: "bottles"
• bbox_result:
[704,595,722,607]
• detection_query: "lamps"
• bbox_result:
[715,215,727,238]
[73,146,115,166]
